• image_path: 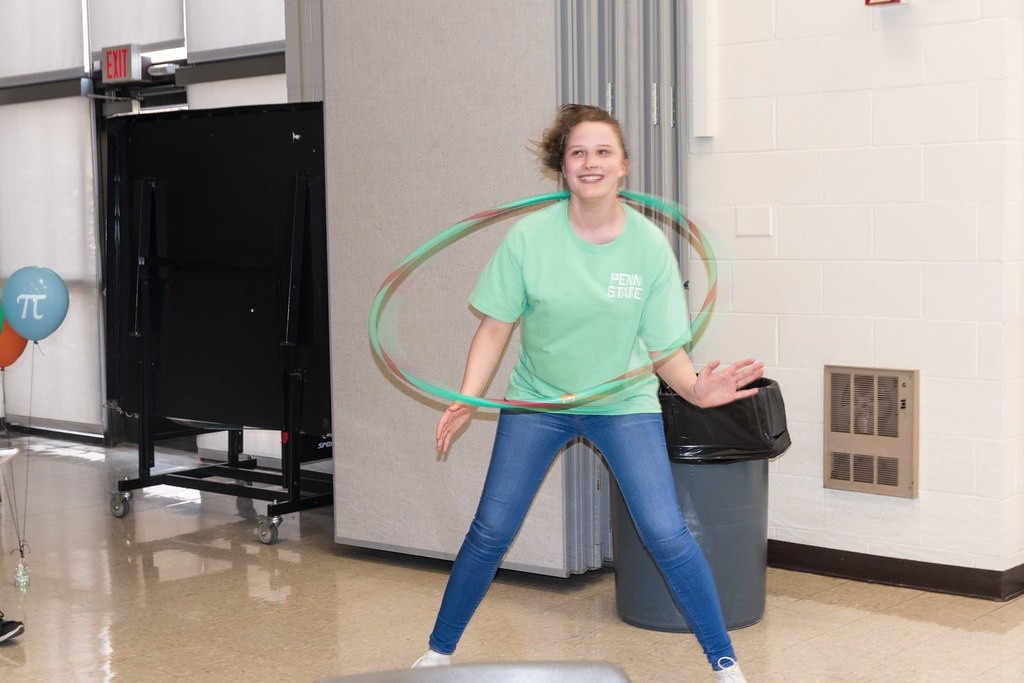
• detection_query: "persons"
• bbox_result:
[412,105,764,683]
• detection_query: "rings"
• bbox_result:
[735,382,740,390]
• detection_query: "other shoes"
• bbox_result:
[715,656,747,683]
[0,610,25,643]
[409,649,452,668]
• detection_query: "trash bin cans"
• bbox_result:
[607,369,791,635]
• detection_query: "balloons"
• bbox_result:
[2,266,69,344]
[0,277,27,373]
